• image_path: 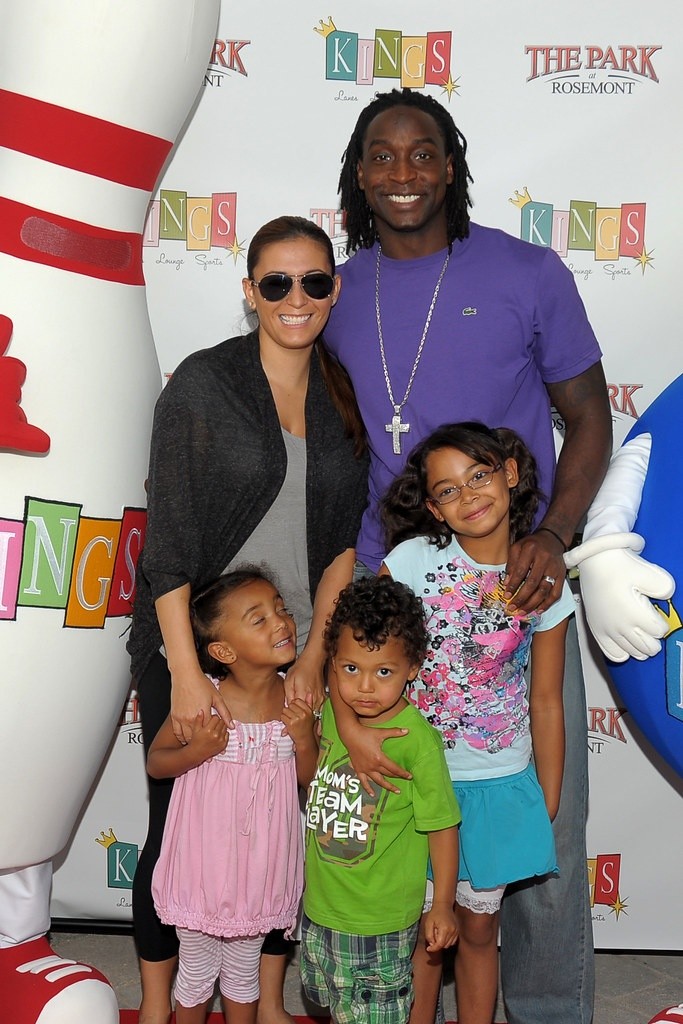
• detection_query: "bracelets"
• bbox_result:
[533,527,568,553]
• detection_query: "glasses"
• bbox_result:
[251,273,335,301]
[430,465,501,505]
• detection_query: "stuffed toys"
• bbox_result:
[0,2,220,1024]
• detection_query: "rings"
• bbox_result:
[312,710,323,720]
[543,575,556,586]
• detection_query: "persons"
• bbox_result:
[562,375,683,1024]
[282,575,464,1024]
[146,562,319,1024]
[127,214,374,1024]
[319,89,613,1024]
[328,419,577,1024]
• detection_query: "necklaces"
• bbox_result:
[375,247,449,455]
